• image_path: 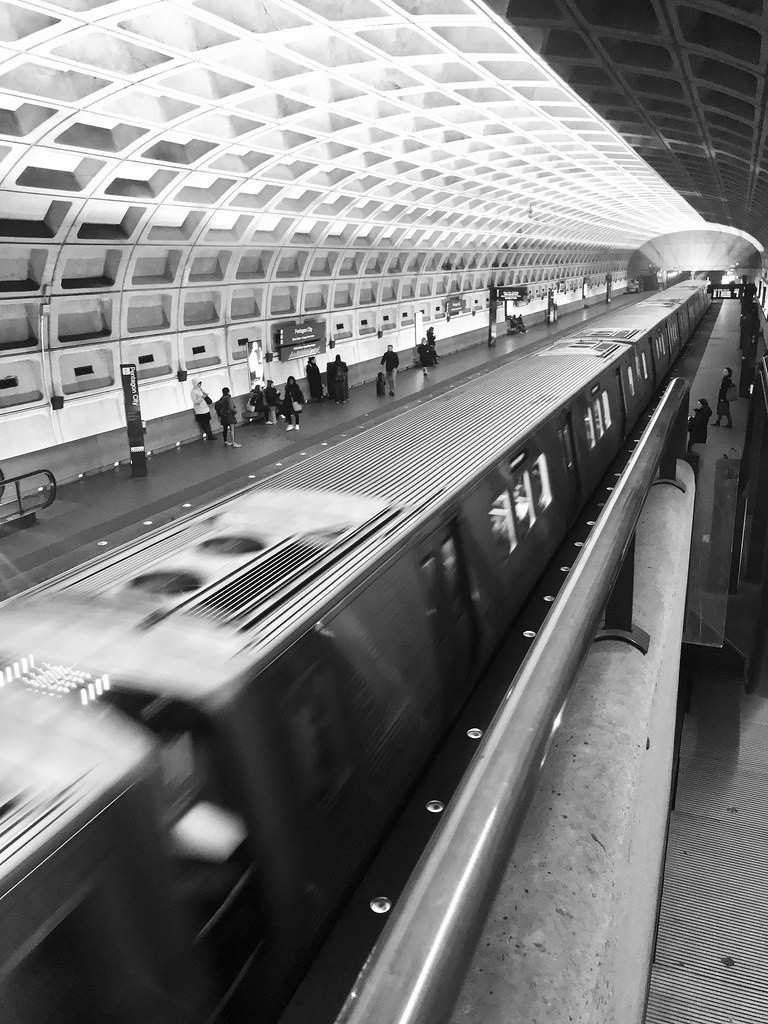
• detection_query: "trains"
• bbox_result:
[0,281,713,1024]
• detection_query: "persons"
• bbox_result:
[306,354,348,403]
[687,399,713,453]
[380,345,399,396]
[417,327,441,376]
[190,378,242,447]
[249,376,304,431]
[710,367,733,428]
[510,314,530,334]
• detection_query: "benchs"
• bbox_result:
[242,406,279,422]
[507,328,517,334]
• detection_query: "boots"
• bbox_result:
[723,421,732,428]
[710,420,720,426]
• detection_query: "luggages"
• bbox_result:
[376,364,385,397]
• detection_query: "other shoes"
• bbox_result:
[526,330,529,333]
[265,421,273,425]
[285,424,299,431]
[388,390,394,396]
[208,436,217,440]
[519,332,523,334]
[278,414,286,419]
[224,441,241,447]
[423,367,427,376]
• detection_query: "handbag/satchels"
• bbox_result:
[726,384,738,402]
[291,401,303,412]
[246,393,258,412]
[203,396,212,405]
